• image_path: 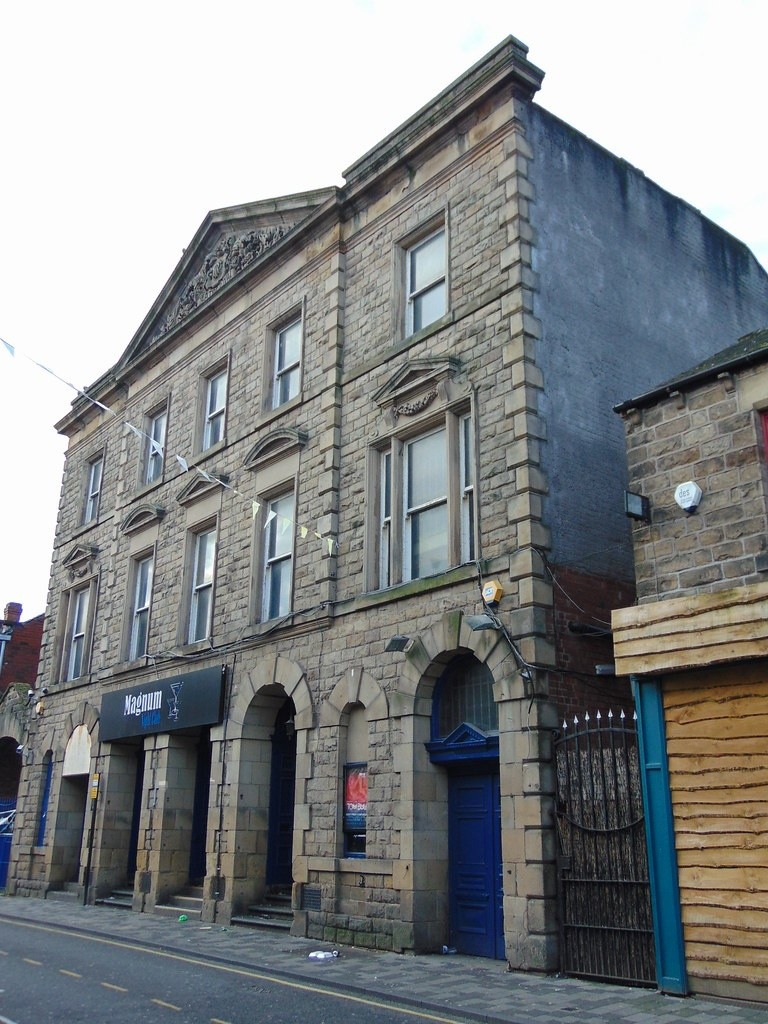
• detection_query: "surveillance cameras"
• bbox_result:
[16,744,25,754]
[28,690,35,697]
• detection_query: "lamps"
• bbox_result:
[385,635,415,653]
[624,490,652,523]
